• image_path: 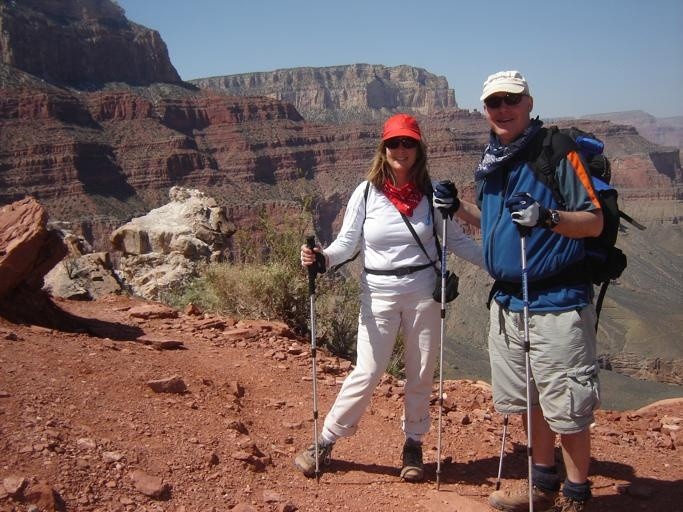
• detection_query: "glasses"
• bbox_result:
[385,137,417,150]
[484,95,520,108]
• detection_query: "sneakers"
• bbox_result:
[402,438,424,480]
[296,434,334,477]
[488,480,560,510]
[560,495,594,512]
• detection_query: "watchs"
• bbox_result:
[548,208,561,229]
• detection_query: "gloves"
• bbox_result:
[505,193,547,229]
[432,179,461,215]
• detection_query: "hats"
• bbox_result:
[480,72,530,100]
[381,115,422,142]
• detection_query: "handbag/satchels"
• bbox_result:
[434,269,460,303]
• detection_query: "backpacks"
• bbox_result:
[528,125,628,285]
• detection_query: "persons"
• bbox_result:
[433,70,604,511]
[295,113,486,484]
